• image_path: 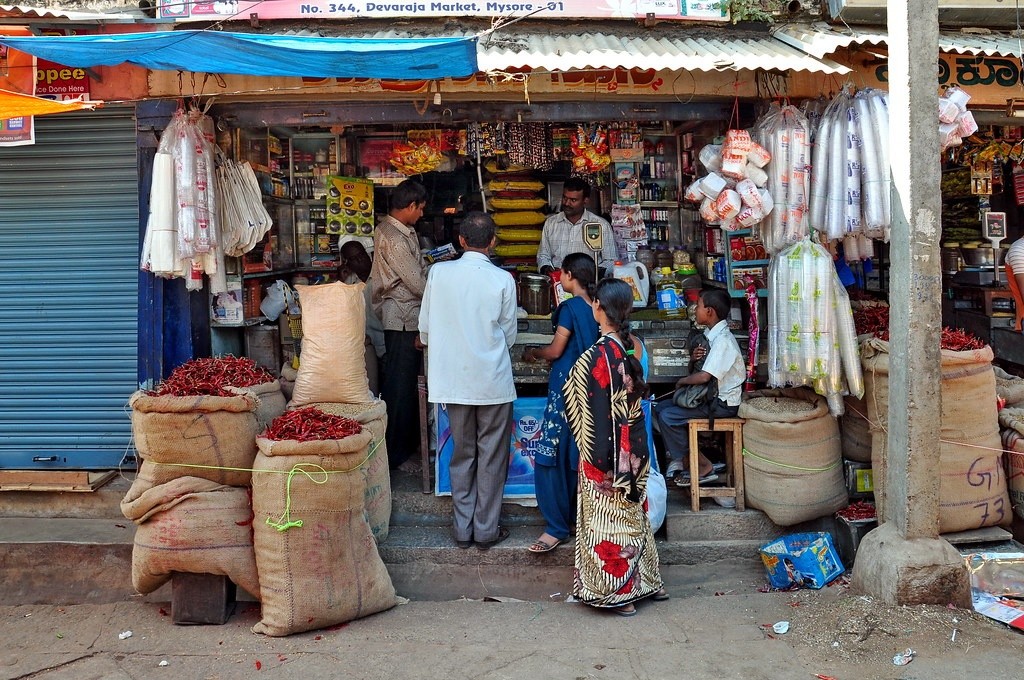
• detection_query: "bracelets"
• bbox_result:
[530,347,537,361]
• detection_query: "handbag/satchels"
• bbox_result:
[647,467,667,535]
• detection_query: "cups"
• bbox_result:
[755,89,890,417]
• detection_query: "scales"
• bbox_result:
[951,211,1009,287]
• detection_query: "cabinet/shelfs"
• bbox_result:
[609,122,770,298]
[941,166,977,200]
[213,127,346,328]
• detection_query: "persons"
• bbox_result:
[521,252,599,552]
[334,179,454,474]
[536,178,619,306]
[654,288,746,488]
[560,278,670,617]
[413,210,517,551]
[784,558,816,589]
[1005,236,1024,335]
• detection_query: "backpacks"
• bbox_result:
[673,329,720,413]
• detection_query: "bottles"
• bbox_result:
[253,136,284,174]
[243,272,339,318]
[941,238,1012,276]
[640,138,677,201]
[641,208,669,241]
[309,208,327,233]
[313,140,336,176]
[294,177,314,199]
[518,273,551,315]
[271,177,289,197]
[991,158,1024,206]
[613,245,729,321]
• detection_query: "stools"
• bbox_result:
[684,417,746,513]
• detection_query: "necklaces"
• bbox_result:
[606,330,617,335]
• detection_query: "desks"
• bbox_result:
[971,286,1014,317]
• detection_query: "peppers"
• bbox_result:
[257,406,362,444]
[256,659,261,670]
[235,485,255,525]
[845,288,984,351]
[836,497,877,520]
[141,354,276,398]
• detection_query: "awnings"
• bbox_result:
[0,21,1024,122]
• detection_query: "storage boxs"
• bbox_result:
[706,228,767,290]
[279,313,294,344]
[757,532,846,590]
[241,230,273,319]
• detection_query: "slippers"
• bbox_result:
[611,602,636,616]
[650,587,669,599]
[674,469,718,486]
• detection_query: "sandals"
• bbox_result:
[570,525,575,534]
[528,533,570,552]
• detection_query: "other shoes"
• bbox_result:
[456,534,473,547]
[476,528,509,549]
